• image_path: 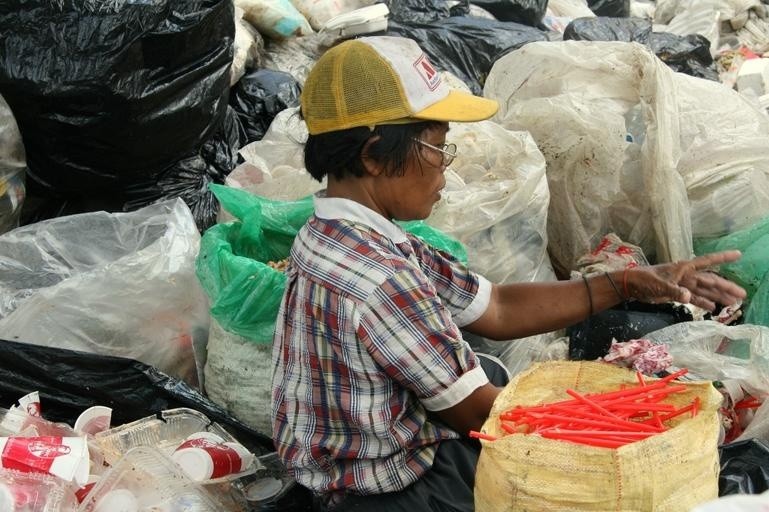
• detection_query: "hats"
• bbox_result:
[300,36,499,135]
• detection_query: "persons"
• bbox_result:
[267,35,749,511]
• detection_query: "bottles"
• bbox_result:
[623,103,645,144]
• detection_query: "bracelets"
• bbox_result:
[604,270,625,303]
[580,276,594,318]
[622,264,631,300]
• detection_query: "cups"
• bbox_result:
[172,431,256,482]
[1,435,91,489]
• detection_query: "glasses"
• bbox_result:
[411,136,457,166]
